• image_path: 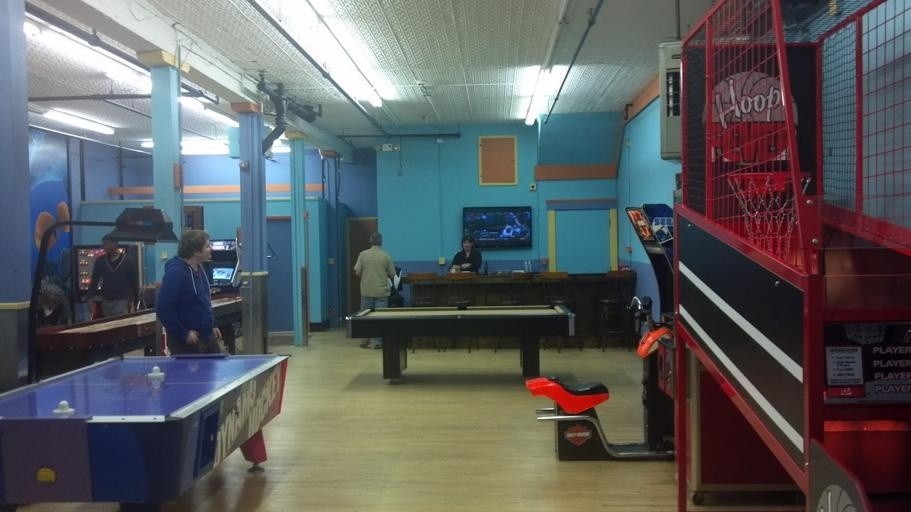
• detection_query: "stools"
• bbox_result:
[599,297,630,351]
[388,295,404,308]
[494,298,523,353]
[408,298,441,353]
[444,298,479,353]
[545,297,583,353]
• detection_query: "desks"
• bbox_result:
[0,355,288,512]
[348,304,577,384]
[39,293,242,374]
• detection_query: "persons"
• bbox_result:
[353,232,396,349]
[35,261,74,327]
[447,235,482,275]
[155,229,223,356]
[85,233,139,318]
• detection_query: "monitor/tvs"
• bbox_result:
[463,206,532,248]
[212,267,234,279]
